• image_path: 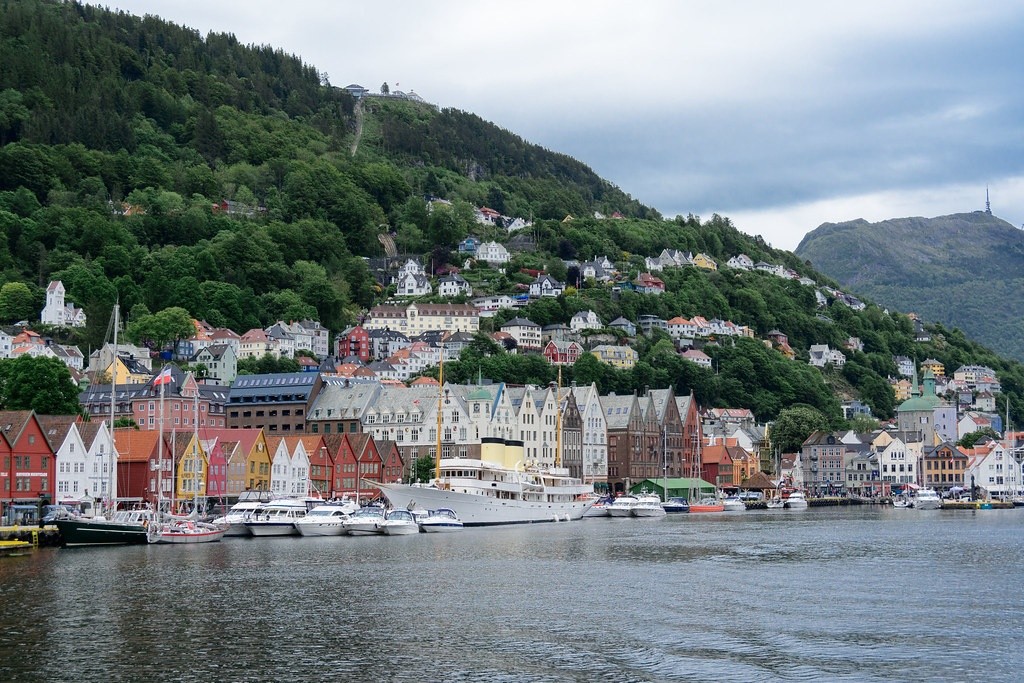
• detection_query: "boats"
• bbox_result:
[344,507,386,536]
[723,500,747,511]
[784,492,808,508]
[380,509,420,535]
[576,494,610,517]
[765,498,786,509]
[893,397,1024,509]
[355,455,600,524]
[420,507,466,531]
[52,300,354,545]
[606,497,639,517]
[630,497,667,519]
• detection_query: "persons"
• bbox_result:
[936,489,942,498]
[811,489,915,499]
[16,510,31,525]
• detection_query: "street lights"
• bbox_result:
[321,446,330,501]
[680,458,686,478]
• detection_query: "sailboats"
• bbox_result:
[652,409,725,513]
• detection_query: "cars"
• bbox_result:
[368,499,385,508]
[726,496,743,502]
[333,500,362,510]
[740,493,748,500]
[748,492,763,500]
